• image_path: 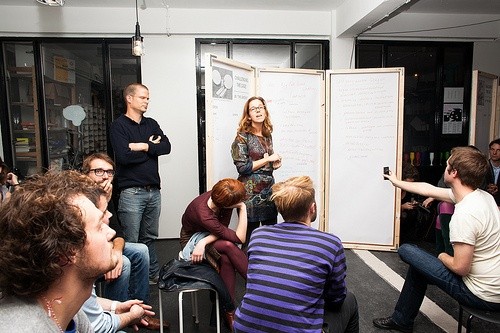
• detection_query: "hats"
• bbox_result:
[0,157,4,166]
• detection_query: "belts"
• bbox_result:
[253,169,272,175]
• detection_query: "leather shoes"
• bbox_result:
[131,316,170,329]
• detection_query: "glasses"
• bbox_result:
[88,168,116,176]
[248,104,265,112]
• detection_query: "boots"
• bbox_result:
[225,309,236,333]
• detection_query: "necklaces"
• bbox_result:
[42,295,64,333]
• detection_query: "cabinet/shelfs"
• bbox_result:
[0,36,107,175]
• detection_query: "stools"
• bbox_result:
[457,302,500,333]
[159,288,220,333]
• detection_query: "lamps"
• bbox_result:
[132,0,144,56]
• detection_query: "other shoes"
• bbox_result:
[149,275,160,284]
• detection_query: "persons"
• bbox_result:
[0,159,155,333]
[179,179,250,331]
[401,139,500,242]
[231,96,282,251]
[372,147,500,333]
[233,176,360,333]
[109,84,172,243]
[77,152,173,331]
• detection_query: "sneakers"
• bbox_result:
[373,316,416,333]
